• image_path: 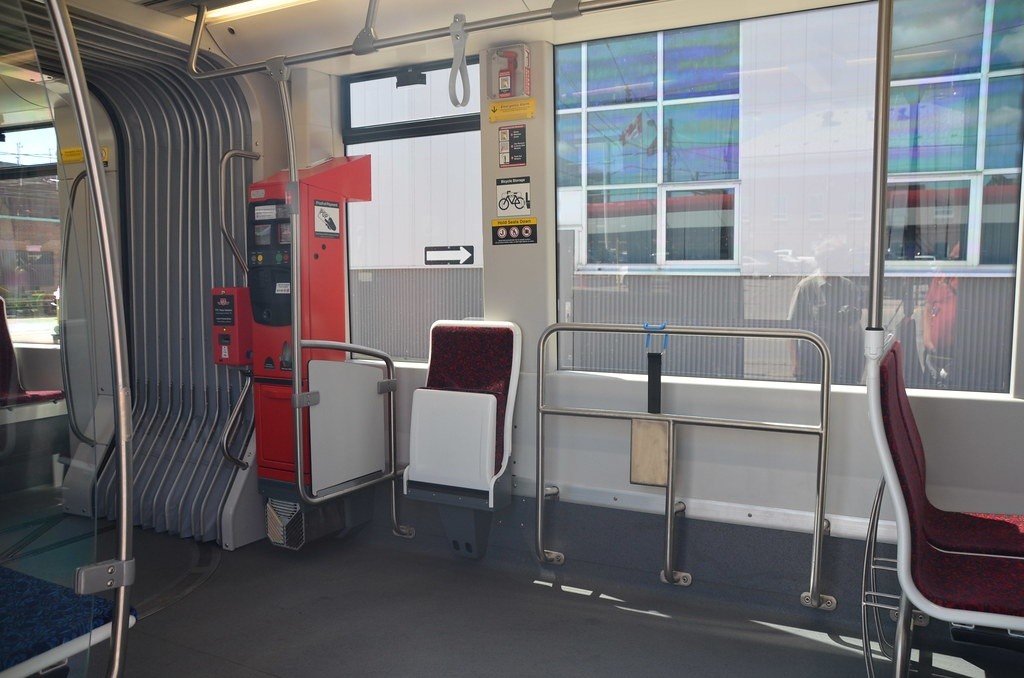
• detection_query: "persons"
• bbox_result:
[785,240,864,387]
[920,241,960,391]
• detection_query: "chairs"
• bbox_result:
[860,332,1024,678]
[402,316,523,564]
[0,296,70,462]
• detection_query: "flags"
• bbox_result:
[647,128,668,155]
[619,113,642,146]
